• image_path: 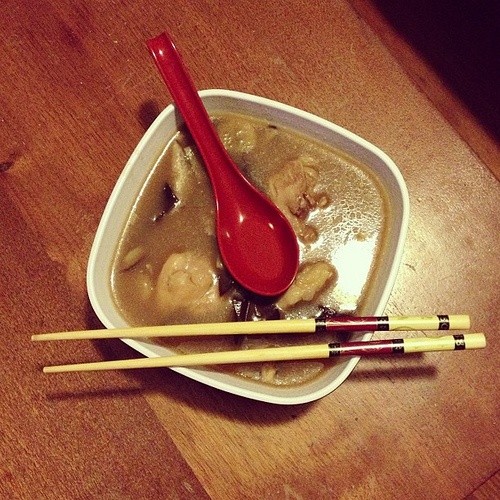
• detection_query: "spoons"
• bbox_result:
[148,32,299,295]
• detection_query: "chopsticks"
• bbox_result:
[32,314,486,373]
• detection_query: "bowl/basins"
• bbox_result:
[87,90,410,405]
